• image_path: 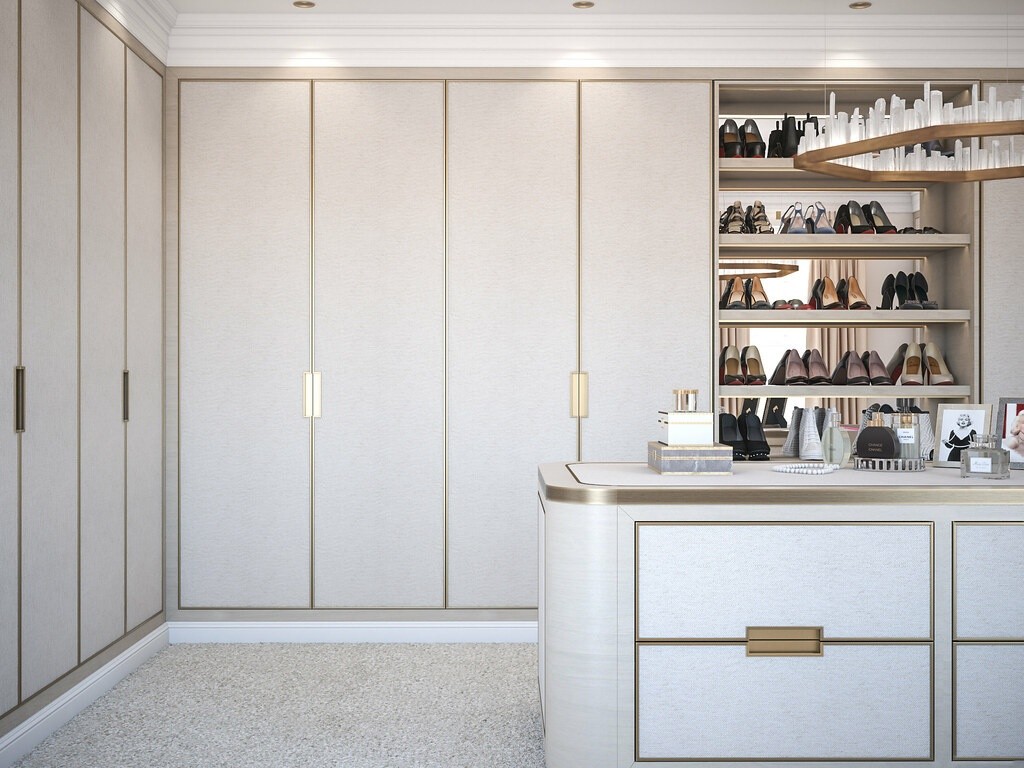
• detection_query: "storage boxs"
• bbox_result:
[658,411,718,447]
[649,443,734,476]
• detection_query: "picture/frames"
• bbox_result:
[933,403,991,467]
[994,396,1024,469]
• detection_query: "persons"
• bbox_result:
[1003,410,1024,458]
[942,413,977,461]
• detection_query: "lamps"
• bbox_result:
[791,82,1024,186]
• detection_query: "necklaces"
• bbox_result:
[772,463,838,474]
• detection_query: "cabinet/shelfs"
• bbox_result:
[78,11,169,657]
[1,3,76,717]
[449,77,708,611]
[712,76,981,438]
[174,72,447,606]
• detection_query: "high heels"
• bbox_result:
[718,111,958,462]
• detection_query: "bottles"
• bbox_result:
[960,433,1011,479]
[867,411,920,458]
[821,412,852,469]
